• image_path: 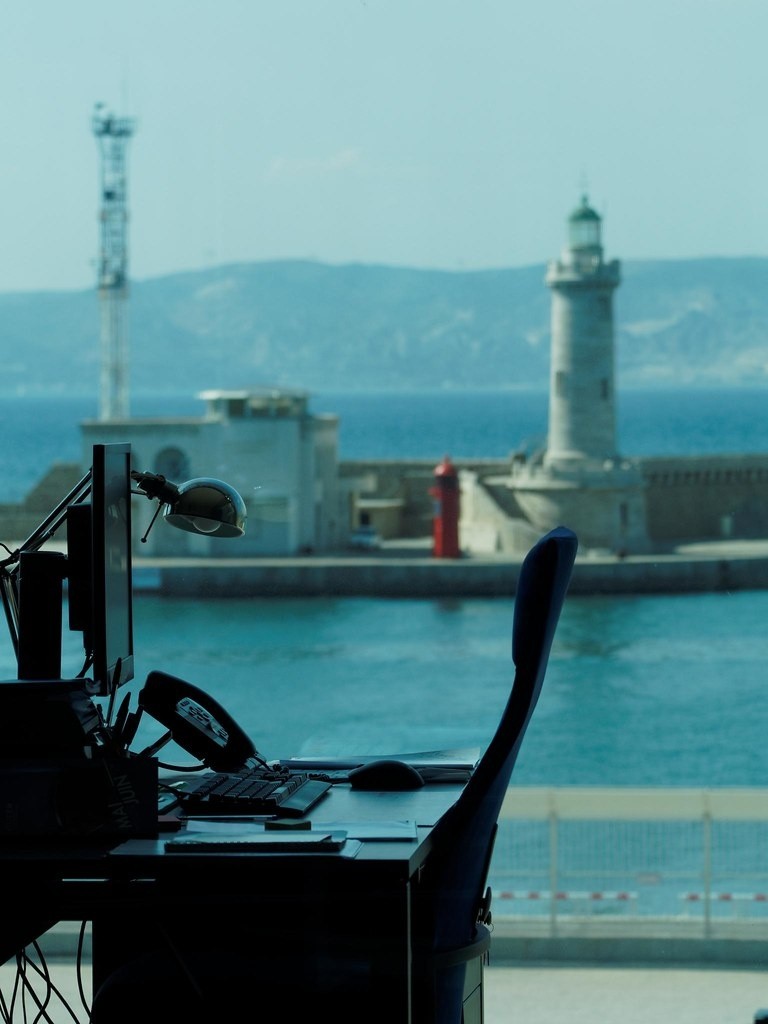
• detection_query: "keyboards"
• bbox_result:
[179,769,332,818]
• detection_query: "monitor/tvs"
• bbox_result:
[66,442,135,697]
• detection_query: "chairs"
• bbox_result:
[415,528,579,1024]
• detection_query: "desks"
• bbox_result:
[90,757,496,1024]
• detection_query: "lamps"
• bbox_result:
[0,462,249,660]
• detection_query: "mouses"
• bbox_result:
[348,760,424,791]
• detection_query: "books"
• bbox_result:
[164,830,348,852]
